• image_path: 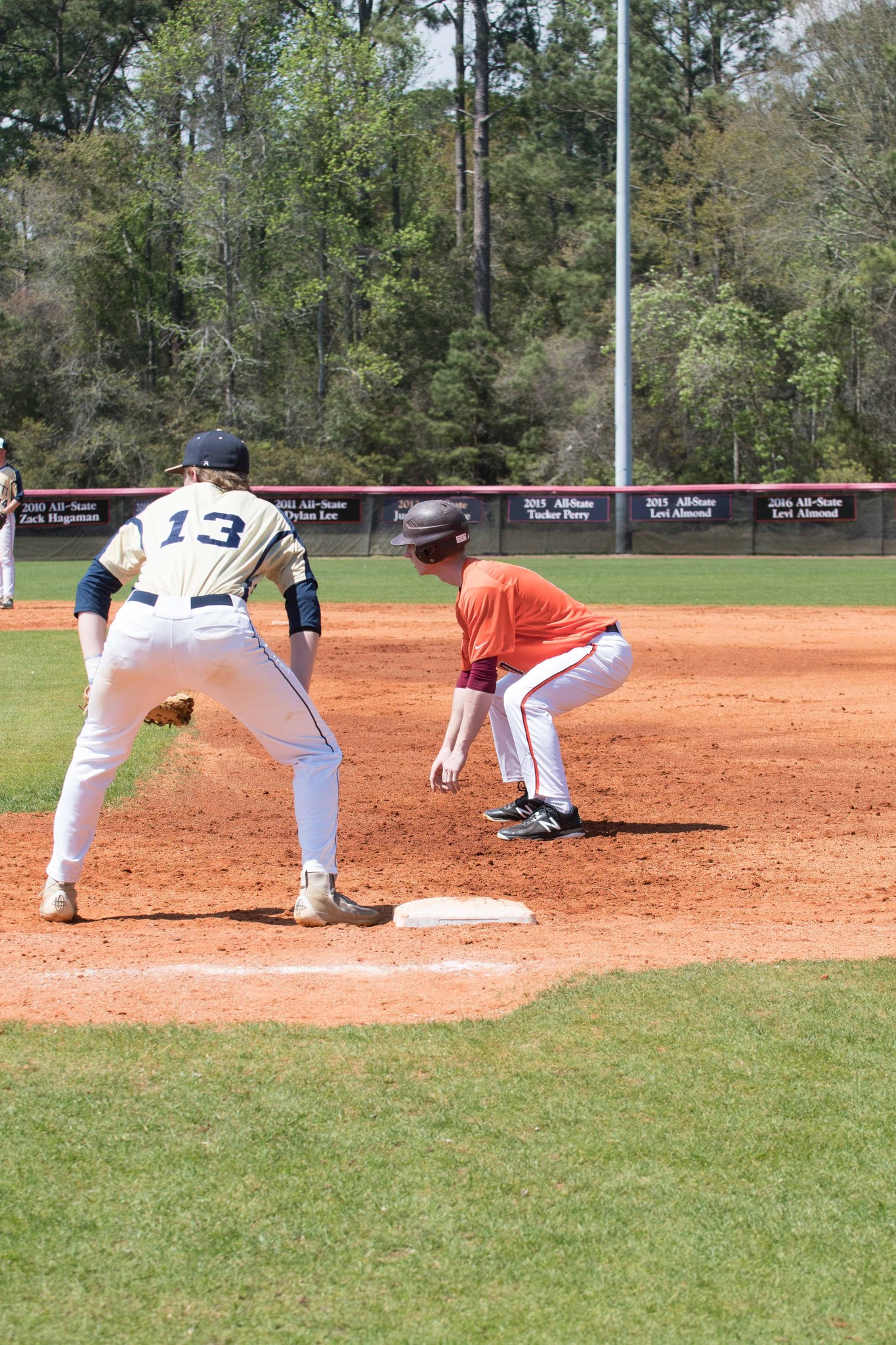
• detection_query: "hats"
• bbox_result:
[165,429,250,474]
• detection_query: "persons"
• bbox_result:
[38,429,379,928]
[390,501,633,840]
[0,438,25,609]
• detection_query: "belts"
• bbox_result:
[128,591,233,609]
[605,623,620,633]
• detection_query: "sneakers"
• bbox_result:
[0,598,14,609]
[483,781,545,822]
[497,803,585,841]
[294,870,381,926]
[38,876,78,922]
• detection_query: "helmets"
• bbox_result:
[390,500,471,564]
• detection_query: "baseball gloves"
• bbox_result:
[76,683,196,731]
